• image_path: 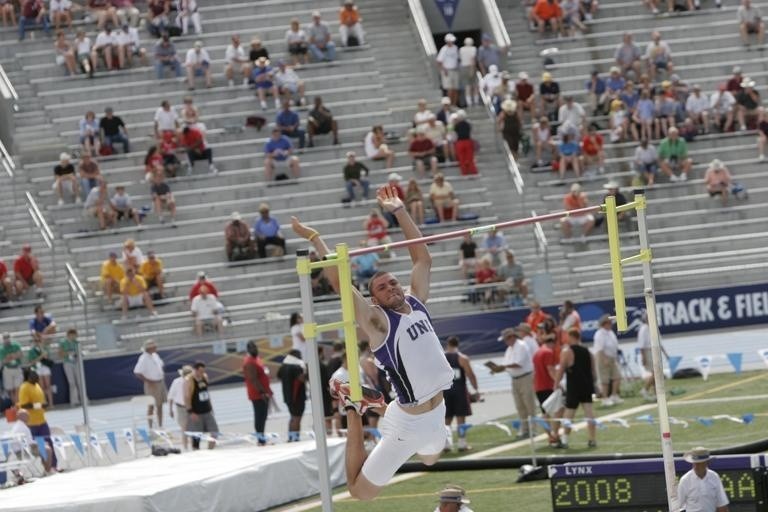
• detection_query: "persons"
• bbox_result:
[290,187,456,502]
[52,96,215,229]
[1,0,364,111]
[678,446,730,511]
[436,227,672,450]
[0,237,388,488]
[222,95,475,265]
[436,1,768,245]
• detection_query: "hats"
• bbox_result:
[683,447,713,463]
[602,181,619,189]
[60,153,69,160]
[444,484,465,498]
[435,489,469,503]
[387,97,466,186]
[231,211,240,222]
[708,159,722,170]
[193,0,353,68]
[177,366,194,379]
[495,309,613,342]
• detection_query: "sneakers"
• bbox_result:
[443,428,598,452]
[338,383,385,407]
[598,388,656,407]
[329,379,368,416]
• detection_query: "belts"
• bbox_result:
[512,371,532,379]
